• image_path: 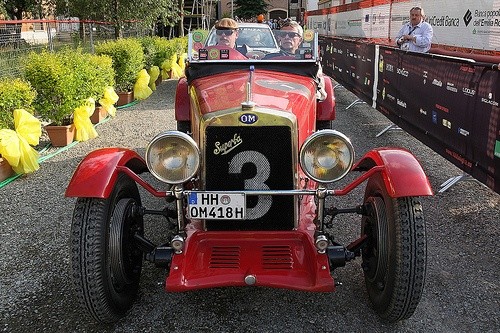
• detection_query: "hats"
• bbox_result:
[215,18,239,29]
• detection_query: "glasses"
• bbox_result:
[216,29,234,36]
[279,32,301,38]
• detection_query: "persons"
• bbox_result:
[204,18,247,57]
[226,17,297,28]
[263,21,307,60]
[394,7,433,54]
[251,34,264,47]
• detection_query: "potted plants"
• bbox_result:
[0,36,188,183]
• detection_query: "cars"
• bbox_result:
[63,29,434,325]
[203,22,281,53]
[54,30,77,44]
